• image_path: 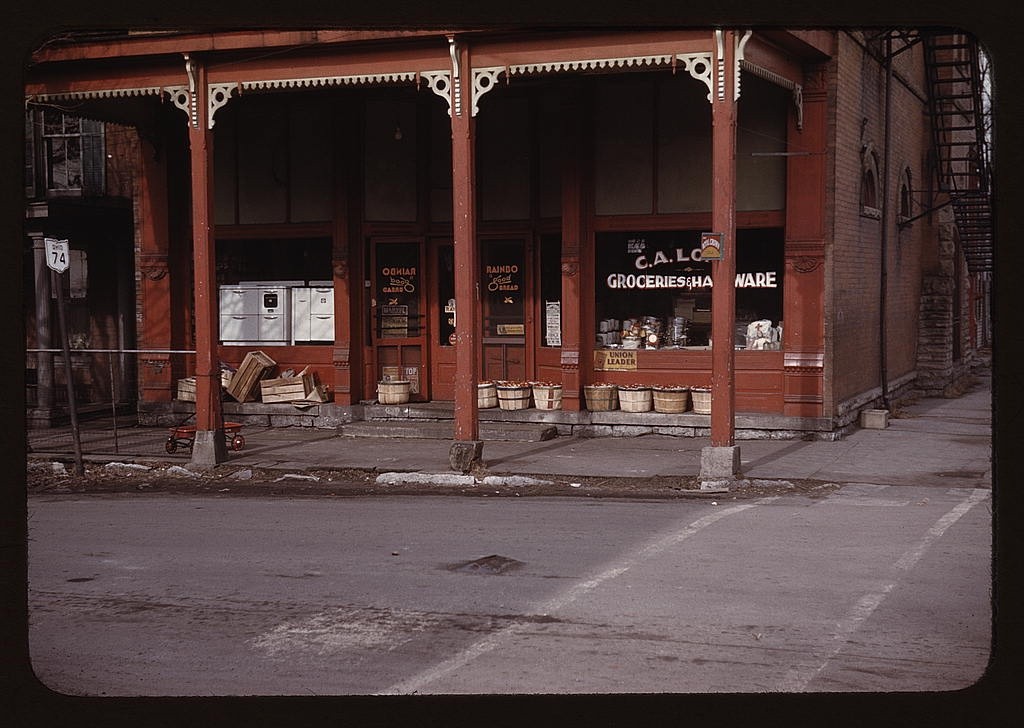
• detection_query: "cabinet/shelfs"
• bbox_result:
[220,285,335,346]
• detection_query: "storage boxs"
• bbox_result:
[260,374,316,403]
[226,351,276,403]
[177,378,197,402]
